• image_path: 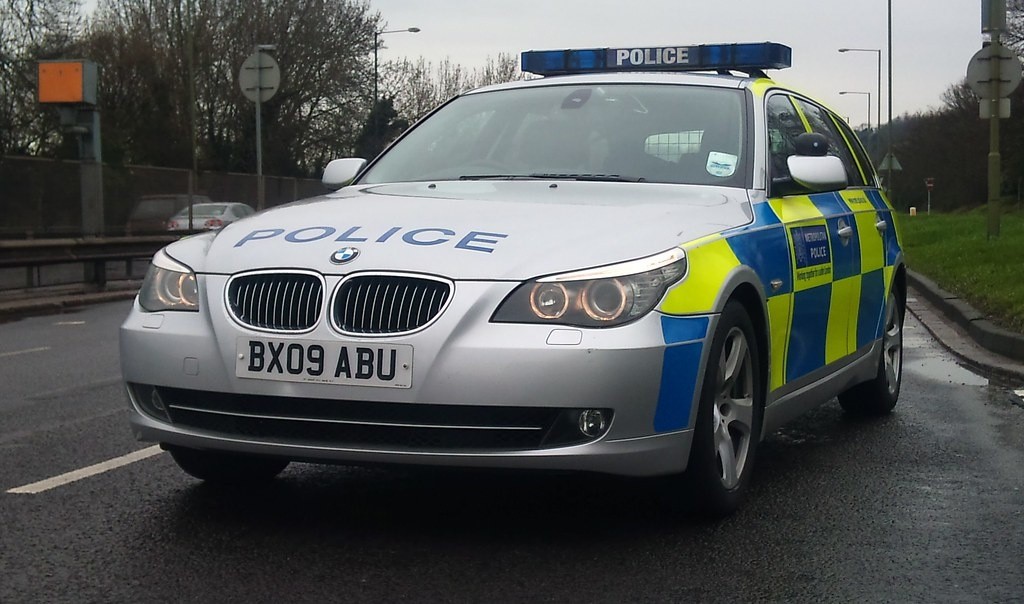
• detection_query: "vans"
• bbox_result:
[129,195,213,235]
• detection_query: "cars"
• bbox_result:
[172,202,255,232]
[119,40,913,510]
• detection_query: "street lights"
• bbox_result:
[839,91,871,148]
[371,27,421,129]
[839,48,882,151]
